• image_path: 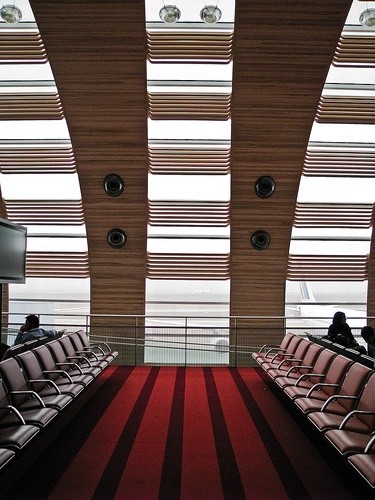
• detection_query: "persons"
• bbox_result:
[13,315,66,347]
[328,311,366,355]
[361,326,375,358]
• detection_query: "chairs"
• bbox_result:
[283,355,353,400]
[1,343,25,360]
[1,356,76,411]
[56,335,109,370]
[7,350,87,399]
[294,362,372,417]
[322,338,332,348]
[346,451,375,489]
[43,340,102,378]
[0,445,16,472]
[361,354,375,366]
[23,338,39,349]
[274,347,338,389]
[304,331,312,339]
[56,328,68,335]
[39,336,50,342]
[308,369,374,433]
[322,426,374,454]
[0,404,41,449]
[261,339,312,372]
[76,329,118,358]
[256,336,303,365]
[1,378,60,428]
[313,335,322,343]
[267,343,324,380]
[346,348,360,361]
[32,344,93,387]
[67,332,114,363]
[332,342,345,354]
[251,332,295,359]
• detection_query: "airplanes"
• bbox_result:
[285,281,367,327]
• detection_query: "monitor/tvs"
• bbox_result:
[0,217,28,284]
